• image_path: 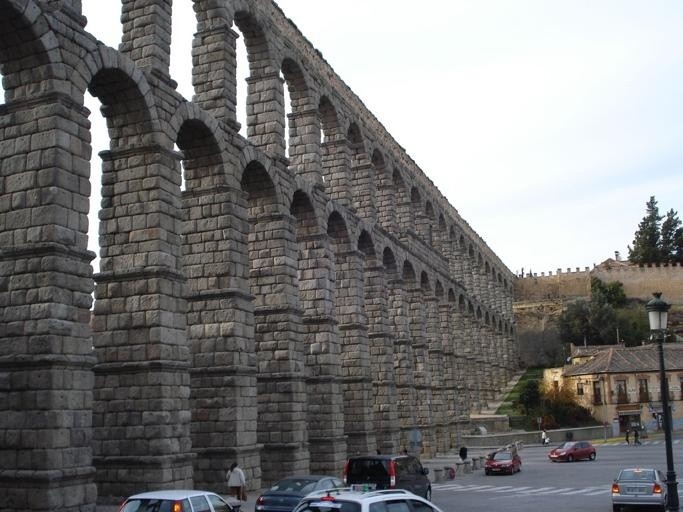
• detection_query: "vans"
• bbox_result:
[343,454,434,502]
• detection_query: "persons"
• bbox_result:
[399,446,407,455]
[458,444,467,461]
[541,430,546,444]
[631,427,641,446]
[224,462,246,501]
[544,437,549,445]
[508,445,514,455]
[376,449,381,455]
[624,429,629,444]
[512,444,517,455]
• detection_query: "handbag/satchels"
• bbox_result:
[242,488,247,501]
[545,438,550,443]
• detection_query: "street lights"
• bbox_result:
[645,290,679,511]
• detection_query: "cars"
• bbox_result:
[608,465,668,511]
[546,440,598,463]
[481,450,523,476]
